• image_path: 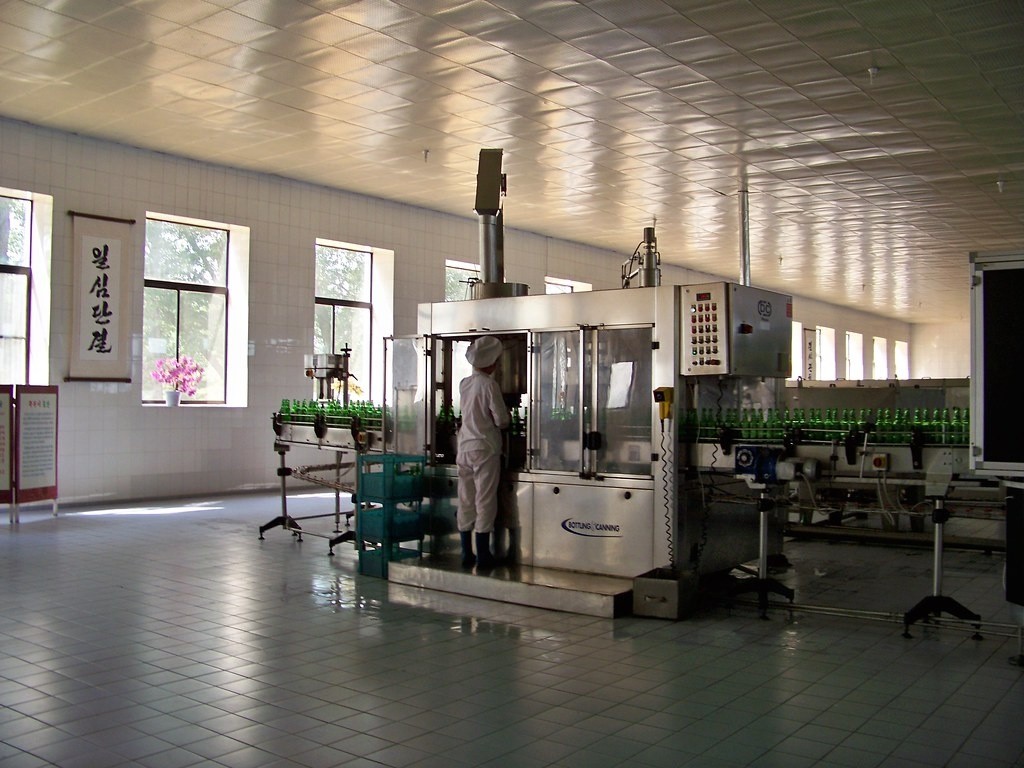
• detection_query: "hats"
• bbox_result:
[465,336,503,368]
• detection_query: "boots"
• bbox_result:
[460,530,476,566]
[475,531,493,569]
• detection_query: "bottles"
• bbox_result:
[414,463,421,477]
[280,398,462,441]
[680,408,970,446]
[513,407,592,435]
[408,465,416,477]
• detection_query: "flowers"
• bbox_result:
[151,356,204,396]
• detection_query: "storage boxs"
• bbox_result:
[634,568,700,619]
[357,453,427,580]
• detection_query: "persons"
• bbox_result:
[458,336,510,568]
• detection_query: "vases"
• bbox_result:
[165,390,180,407]
